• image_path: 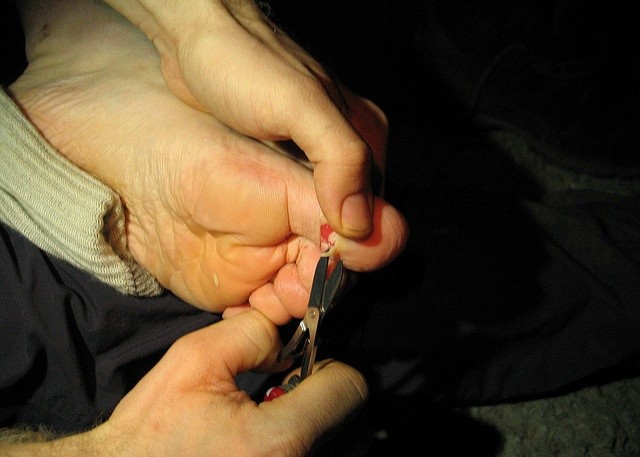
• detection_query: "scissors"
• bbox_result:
[257,253,343,402]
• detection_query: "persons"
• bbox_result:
[0,0,410,456]
